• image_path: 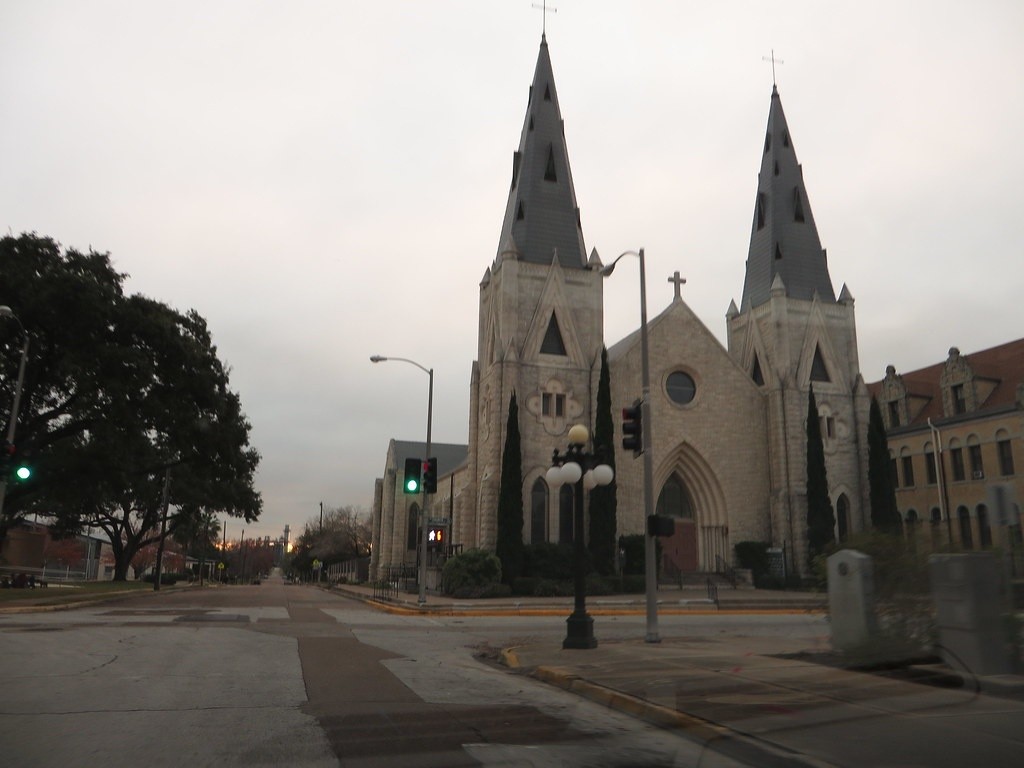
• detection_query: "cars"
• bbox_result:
[254,574,267,585]
[284,580,291,585]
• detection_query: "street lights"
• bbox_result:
[0,305,33,511]
[600,250,662,643]
[369,355,433,603]
[546,424,614,649]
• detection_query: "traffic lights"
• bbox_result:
[423,458,437,495]
[403,457,422,494]
[425,526,444,545]
[12,448,33,480]
[622,400,644,461]
[646,513,674,536]
